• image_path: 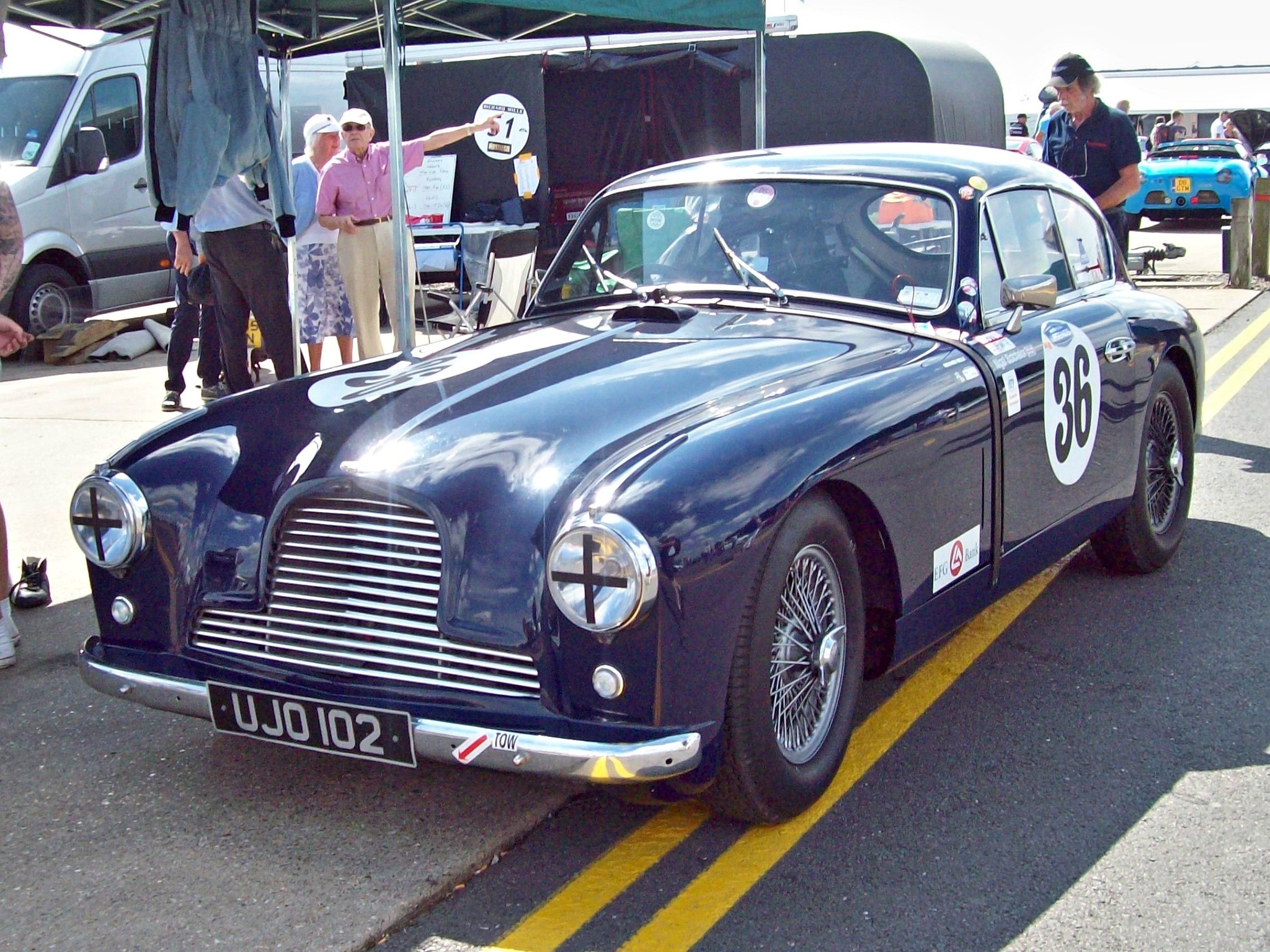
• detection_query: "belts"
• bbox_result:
[243,221,271,230]
[353,214,393,226]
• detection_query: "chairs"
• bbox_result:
[427,227,538,338]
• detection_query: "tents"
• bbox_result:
[0,2,768,352]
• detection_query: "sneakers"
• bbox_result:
[14,556,50,609]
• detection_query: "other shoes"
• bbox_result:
[201,383,230,401]
[161,389,182,412]
[0,595,21,669]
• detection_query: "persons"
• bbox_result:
[315,108,504,361]
[159,173,295,413]
[1040,52,1141,275]
[0,179,35,669]
[291,113,356,373]
[1007,102,1238,160]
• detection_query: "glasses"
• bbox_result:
[342,123,366,132]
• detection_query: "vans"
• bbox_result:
[0,21,356,363]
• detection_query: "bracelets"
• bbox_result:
[464,122,473,137]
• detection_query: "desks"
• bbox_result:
[409,222,540,308]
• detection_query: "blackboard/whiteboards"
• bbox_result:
[405,154,457,224]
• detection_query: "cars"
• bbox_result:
[1124,139,1269,230]
[1005,135,1044,163]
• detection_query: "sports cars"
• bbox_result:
[71,142,1206,832]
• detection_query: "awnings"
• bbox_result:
[1093,65,1270,116]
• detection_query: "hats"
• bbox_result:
[1017,113,1030,119]
[303,114,341,140]
[1047,52,1090,88]
[339,108,373,126]
